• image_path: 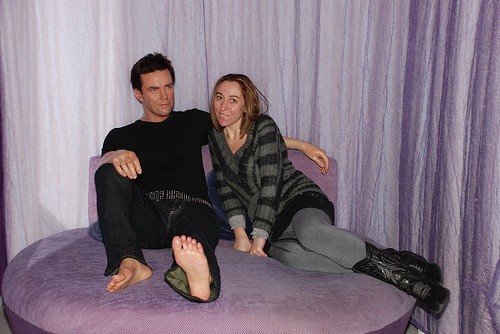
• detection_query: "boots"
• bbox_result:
[352,242,450,315]
[382,248,442,283]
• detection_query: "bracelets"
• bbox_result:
[251,236,268,240]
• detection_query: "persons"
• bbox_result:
[93,51,329,302]
[207,74,451,319]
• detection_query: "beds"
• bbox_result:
[1,146,417,334]
